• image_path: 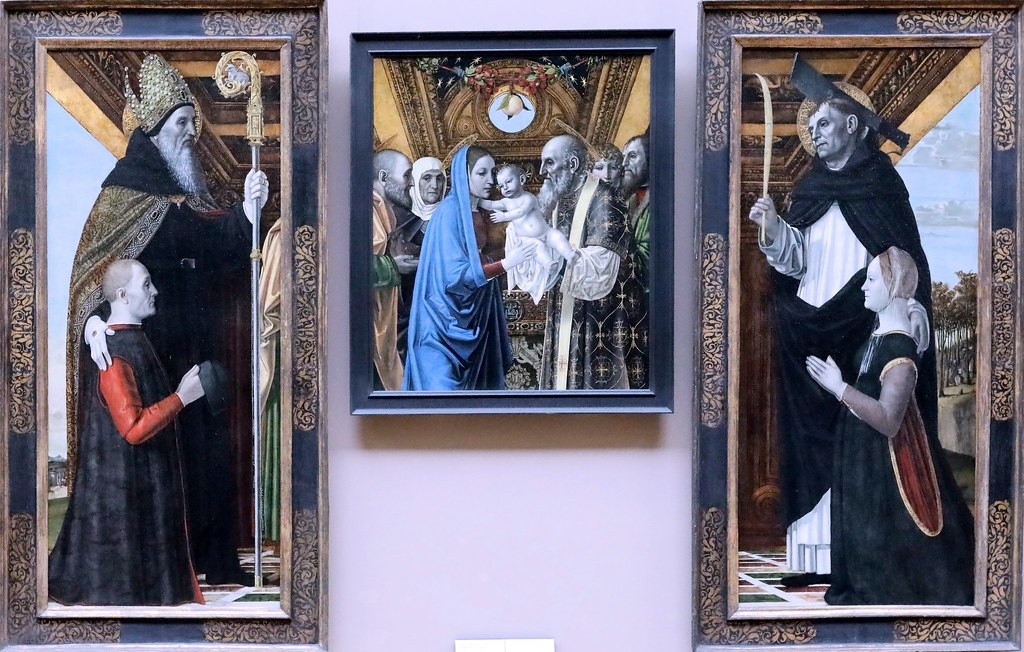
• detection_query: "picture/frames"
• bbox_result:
[691,1,1024,652]
[349,29,675,415]
[0,0,328,652]
